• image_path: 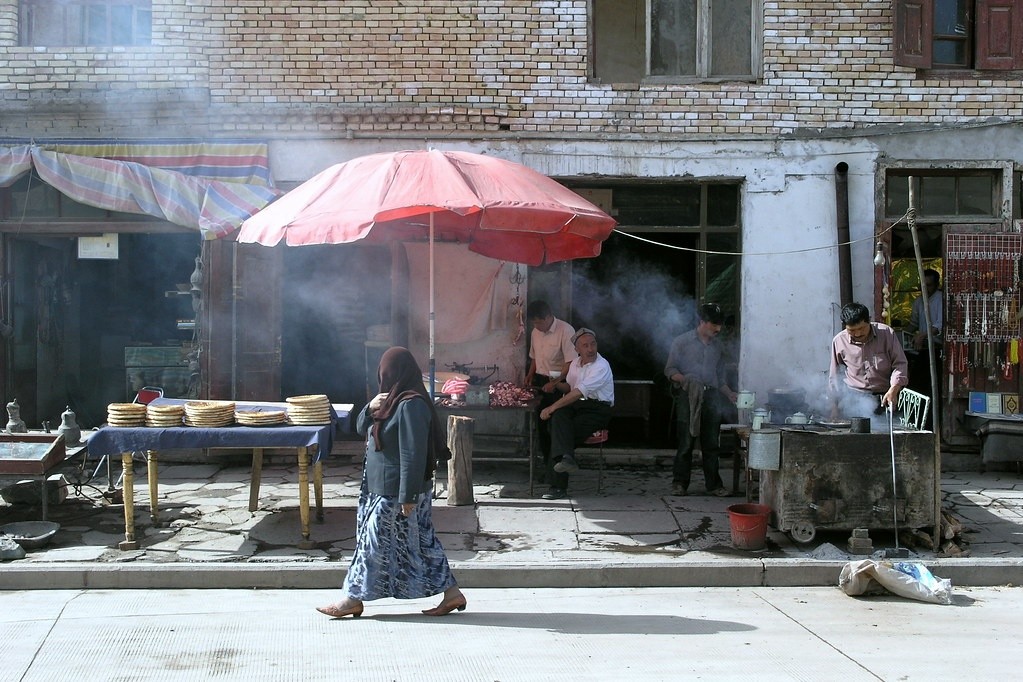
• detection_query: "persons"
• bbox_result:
[909,269,942,436]
[316,346,466,618]
[827,303,909,431]
[664,303,739,497]
[542,328,615,499]
[520,300,579,483]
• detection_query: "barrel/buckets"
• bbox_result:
[727,504,772,550]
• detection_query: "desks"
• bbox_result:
[0,428,115,521]
[87,398,354,542]
[364,340,391,403]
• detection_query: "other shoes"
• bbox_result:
[670,484,686,496]
[541,486,567,500]
[552,454,579,473]
[705,487,734,497]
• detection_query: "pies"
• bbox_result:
[286,394,331,425]
[107,400,235,427]
[233,411,285,425]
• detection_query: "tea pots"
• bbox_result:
[752,414,768,431]
[785,411,806,425]
[733,389,756,408]
[749,406,772,424]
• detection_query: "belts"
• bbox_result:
[703,385,717,390]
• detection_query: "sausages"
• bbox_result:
[441,377,469,393]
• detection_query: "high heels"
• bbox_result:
[422,594,466,615]
[316,601,363,617]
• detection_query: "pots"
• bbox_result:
[766,382,806,406]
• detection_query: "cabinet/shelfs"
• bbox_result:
[125,346,195,404]
[164,291,196,330]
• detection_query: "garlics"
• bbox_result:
[882,287,890,318]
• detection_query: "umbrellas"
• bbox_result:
[237,147,620,399]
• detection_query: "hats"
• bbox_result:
[697,303,724,324]
[570,327,596,345]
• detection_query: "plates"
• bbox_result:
[816,419,851,428]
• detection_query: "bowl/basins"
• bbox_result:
[422,372,470,393]
[0,521,60,550]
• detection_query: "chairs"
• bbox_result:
[92,386,164,486]
